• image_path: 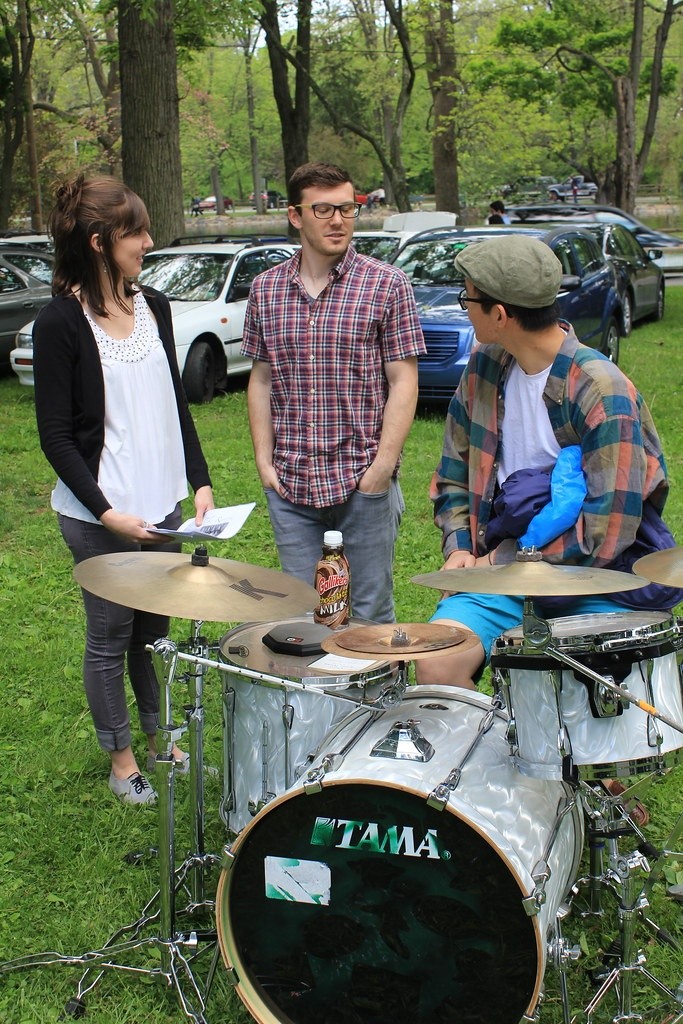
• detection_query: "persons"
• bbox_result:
[366,186,385,209]
[191,191,200,217]
[414,234,670,825]
[570,176,578,203]
[239,161,427,626]
[31,171,219,806]
[488,200,511,224]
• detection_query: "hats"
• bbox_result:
[453,235,562,308]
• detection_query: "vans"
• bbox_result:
[502,175,559,197]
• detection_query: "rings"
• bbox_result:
[144,522,148,528]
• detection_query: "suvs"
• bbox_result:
[483,203,683,280]
[9,232,310,403]
[249,190,288,210]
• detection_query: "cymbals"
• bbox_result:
[321,623,478,662]
[410,561,651,595]
[73,550,322,621]
[633,547,683,590]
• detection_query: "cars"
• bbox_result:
[344,211,668,404]
[0,231,56,378]
[199,194,234,211]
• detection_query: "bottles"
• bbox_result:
[313,530,352,631]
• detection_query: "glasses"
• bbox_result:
[294,201,362,219]
[457,287,513,318]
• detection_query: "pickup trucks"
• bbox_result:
[546,174,598,201]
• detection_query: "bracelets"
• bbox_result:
[489,549,493,565]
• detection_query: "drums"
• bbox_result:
[494,612,683,782]
[218,617,406,833]
[215,685,582,1024]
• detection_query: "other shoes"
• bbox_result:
[604,780,651,827]
[146,752,219,780]
[107,770,156,807]
